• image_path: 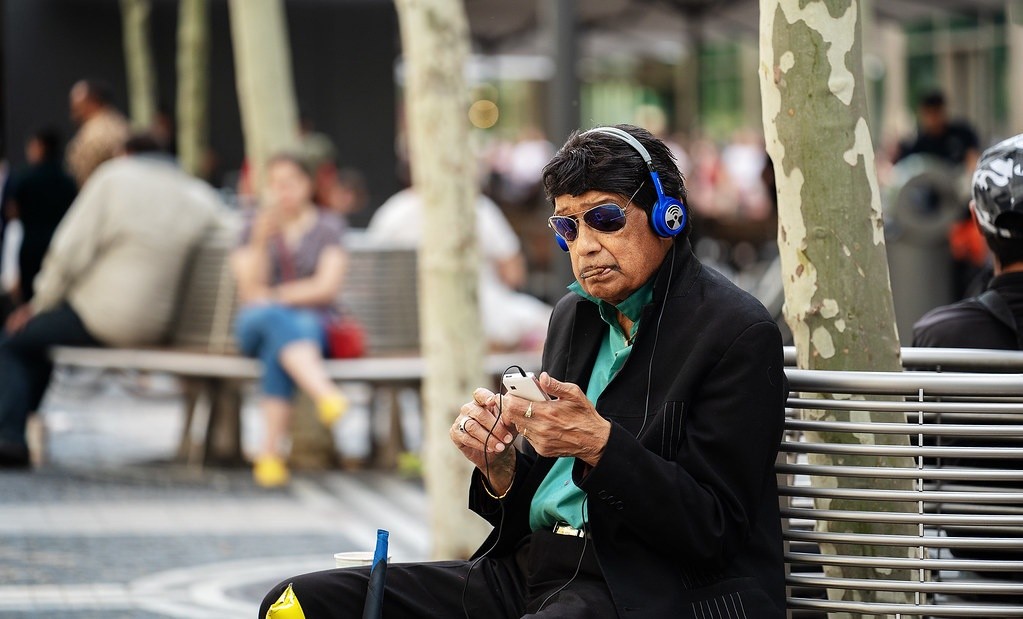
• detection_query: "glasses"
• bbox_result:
[546,182,644,243]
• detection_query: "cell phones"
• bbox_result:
[502,371,551,403]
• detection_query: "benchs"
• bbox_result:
[51,245,540,483]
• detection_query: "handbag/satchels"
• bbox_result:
[329,319,365,360]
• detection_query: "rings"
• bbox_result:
[458,415,473,433]
[524,401,532,417]
[523,428,526,436]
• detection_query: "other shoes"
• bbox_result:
[252,452,292,489]
[317,391,348,426]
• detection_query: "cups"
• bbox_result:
[334,552,392,568]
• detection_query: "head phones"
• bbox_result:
[554,127,687,254]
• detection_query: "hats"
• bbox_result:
[970,134,1023,239]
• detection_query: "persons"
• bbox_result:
[227,148,374,490]
[363,181,556,355]
[877,80,994,299]
[0,74,241,472]
[258,123,788,619]
[898,132,1023,609]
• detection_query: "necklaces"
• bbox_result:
[619,330,637,348]
